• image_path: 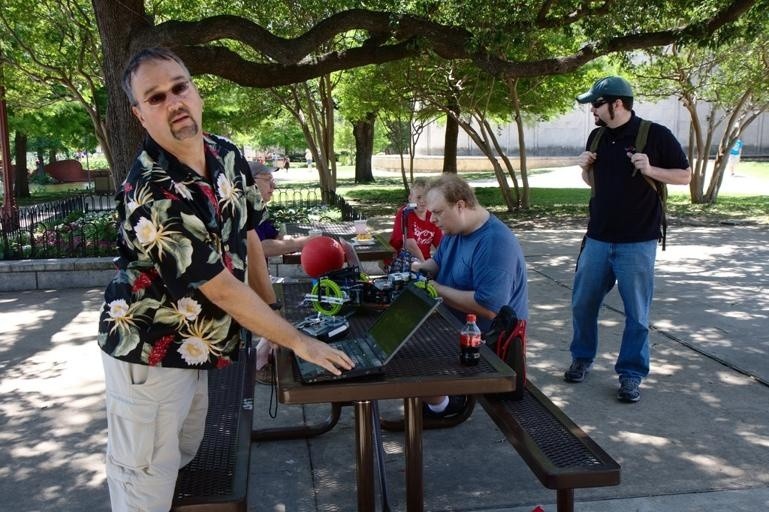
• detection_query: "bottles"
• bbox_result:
[460,314,483,369]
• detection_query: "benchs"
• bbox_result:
[476,371,621,512]
[170,348,257,511]
[238,329,253,350]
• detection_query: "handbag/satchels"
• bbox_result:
[482,305,525,402]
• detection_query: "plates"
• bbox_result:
[350,236,374,243]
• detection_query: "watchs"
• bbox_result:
[269,298,283,310]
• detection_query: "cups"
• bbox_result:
[309,230,322,237]
[355,220,368,240]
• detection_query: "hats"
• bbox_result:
[576,77,632,103]
[248,161,280,178]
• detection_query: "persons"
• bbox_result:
[72,150,87,160]
[410,170,529,421]
[246,161,326,387]
[561,77,693,404]
[95,45,356,512]
[254,151,291,171]
[305,148,313,168]
[728,131,743,176]
[381,178,443,281]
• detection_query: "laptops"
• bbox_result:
[339,236,388,279]
[295,282,440,385]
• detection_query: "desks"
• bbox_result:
[282,221,397,264]
[272,280,517,511]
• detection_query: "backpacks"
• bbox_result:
[588,119,668,225]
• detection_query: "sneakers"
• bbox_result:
[255,363,276,384]
[382,396,466,431]
[617,376,640,401]
[564,358,592,381]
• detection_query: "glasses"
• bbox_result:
[591,100,607,108]
[142,81,190,105]
